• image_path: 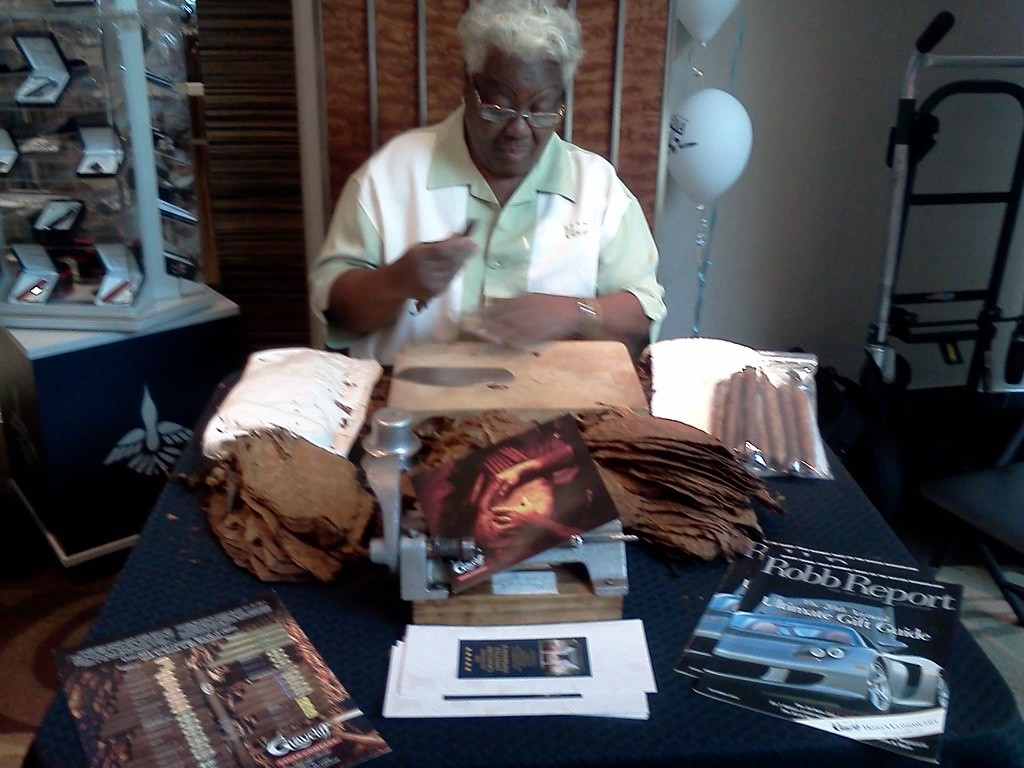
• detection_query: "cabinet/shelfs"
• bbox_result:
[0,0,216,332]
[0,284,239,585]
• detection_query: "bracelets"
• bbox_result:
[576,297,604,344]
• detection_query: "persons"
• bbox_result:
[307,1,669,365]
[480,445,587,540]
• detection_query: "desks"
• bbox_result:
[21,371,1024,768]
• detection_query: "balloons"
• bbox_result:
[670,0,739,46]
[666,88,754,203]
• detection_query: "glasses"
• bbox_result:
[468,75,564,128]
